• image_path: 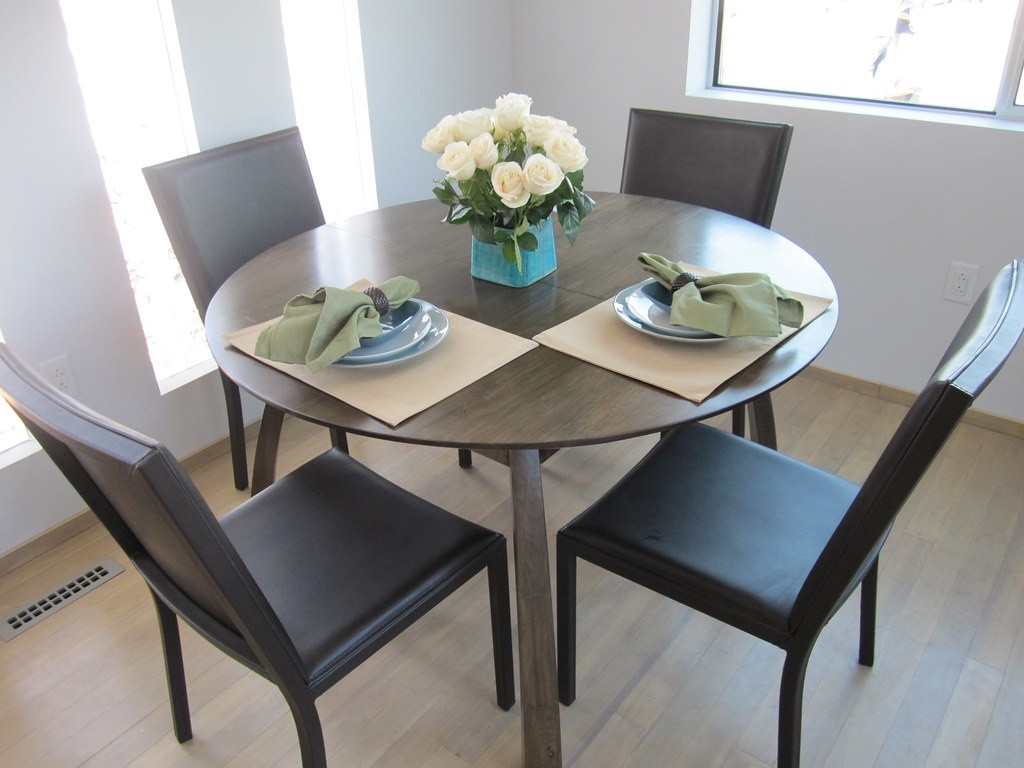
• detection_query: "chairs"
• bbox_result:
[618,107,795,440]
[143,126,471,492]
[1,342,518,768]
[563,262,1024,768]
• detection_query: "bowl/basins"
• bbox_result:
[641,281,672,313]
[360,300,422,347]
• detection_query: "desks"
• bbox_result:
[206,201,840,768]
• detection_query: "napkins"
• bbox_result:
[255,275,420,374]
[531,259,833,403]
[636,252,806,339]
[219,278,539,429]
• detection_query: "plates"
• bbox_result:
[343,309,431,361]
[331,300,450,368]
[613,283,731,342]
[628,289,712,336]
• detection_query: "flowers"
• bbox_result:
[419,90,595,277]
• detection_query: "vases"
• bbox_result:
[469,222,560,287]
